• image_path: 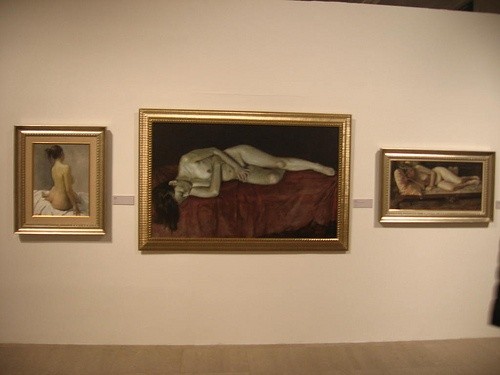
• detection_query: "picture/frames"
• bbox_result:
[380,149,495,227]
[11,124,107,237]
[136,108,352,253]
